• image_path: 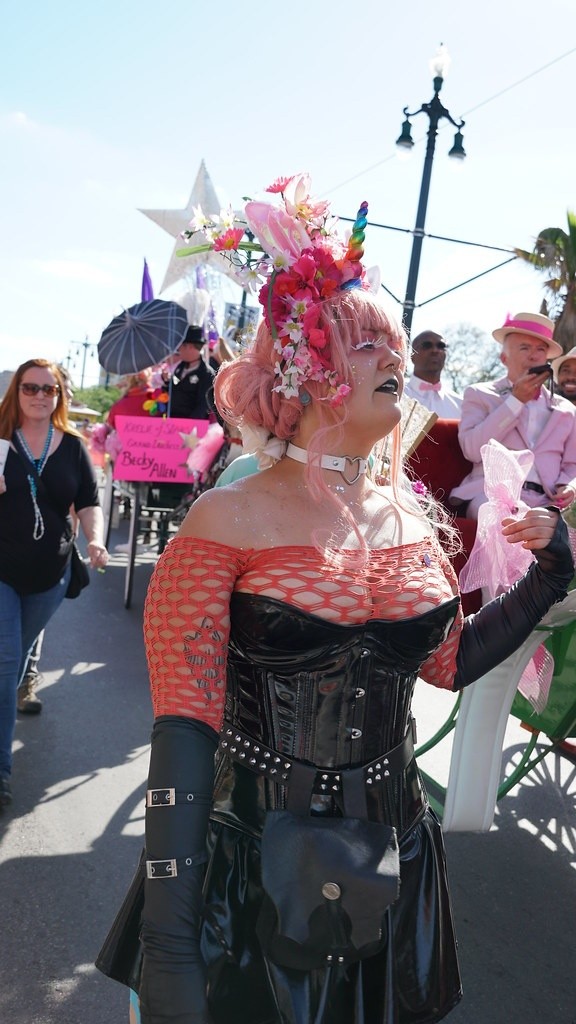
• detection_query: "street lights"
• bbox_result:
[61,333,98,391]
[331,42,519,376]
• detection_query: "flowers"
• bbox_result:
[175,174,380,407]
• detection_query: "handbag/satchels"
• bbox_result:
[256,811,399,972]
[65,545,89,598]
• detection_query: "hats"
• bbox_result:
[183,326,207,344]
[550,346,576,384]
[492,312,563,359]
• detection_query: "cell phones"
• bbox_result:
[528,363,551,375]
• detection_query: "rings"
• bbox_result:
[534,384,538,389]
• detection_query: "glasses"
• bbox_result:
[19,383,59,397]
[415,341,445,349]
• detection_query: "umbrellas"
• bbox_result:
[98,299,189,375]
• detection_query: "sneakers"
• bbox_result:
[16,676,41,713]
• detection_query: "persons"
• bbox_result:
[209,340,221,371]
[404,330,464,420]
[104,368,165,431]
[104,323,217,510]
[449,311,576,517]
[95,286,576,1024]
[0,358,110,806]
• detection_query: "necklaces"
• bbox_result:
[285,444,369,486]
[15,422,55,540]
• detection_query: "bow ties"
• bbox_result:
[419,382,441,391]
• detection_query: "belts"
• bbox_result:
[522,481,545,494]
